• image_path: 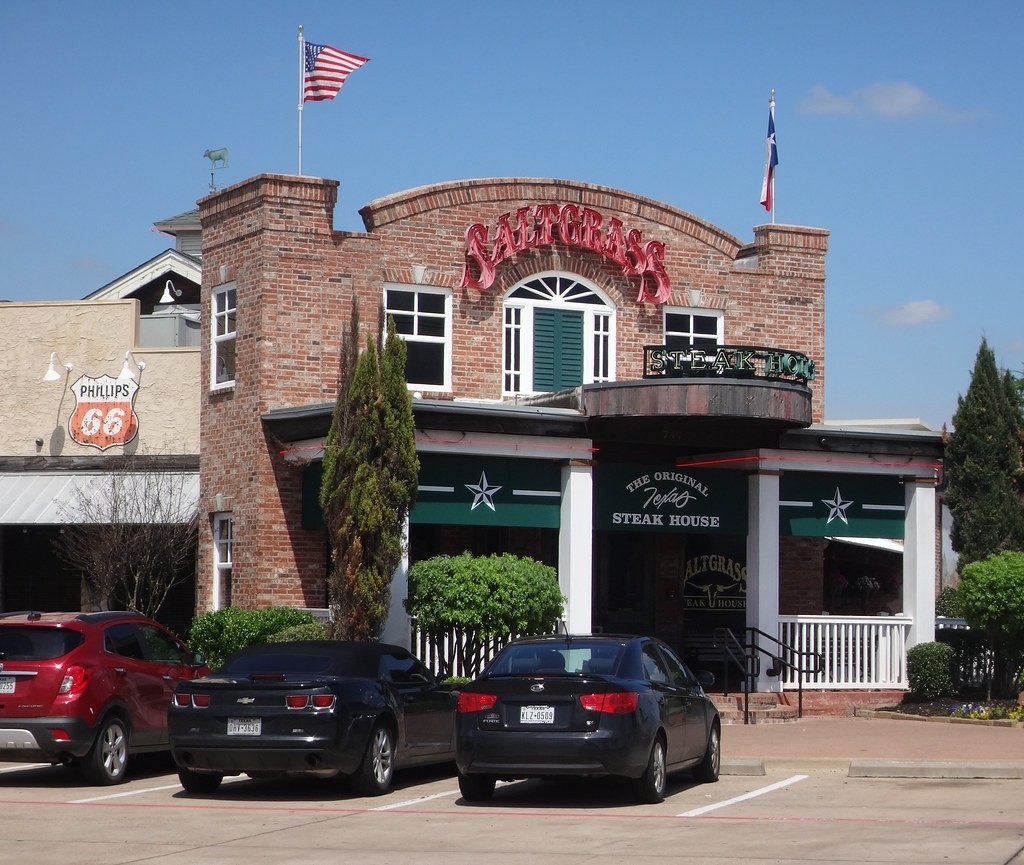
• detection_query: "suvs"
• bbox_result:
[0,610,213,786]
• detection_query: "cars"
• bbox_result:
[454,635,721,801]
[167,641,459,794]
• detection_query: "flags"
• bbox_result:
[760,108,779,212]
[304,41,371,103]
